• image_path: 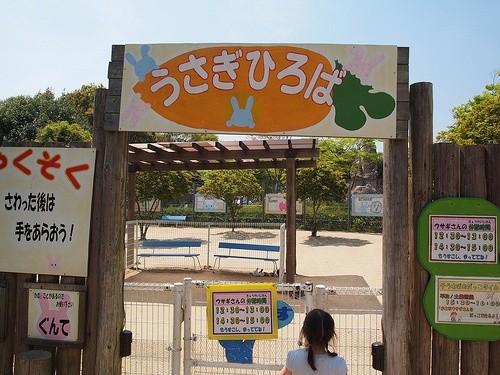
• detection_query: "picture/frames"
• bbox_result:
[205,284,278,341]
[21,283,86,346]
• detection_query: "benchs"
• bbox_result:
[213,242,280,275]
[161,216,186,227]
[137,241,203,273]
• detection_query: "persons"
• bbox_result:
[279,309,349,375]
